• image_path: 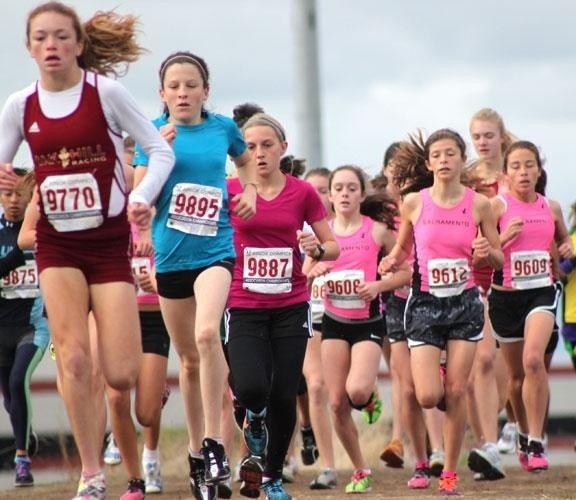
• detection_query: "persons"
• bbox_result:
[300,107,575,494]
[1,4,341,500]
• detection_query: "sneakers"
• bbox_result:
[71,383,170,500]
[189,372,383,499]
[14,426,38,487]
[437,357,447,412]
[379,421,548,495]
